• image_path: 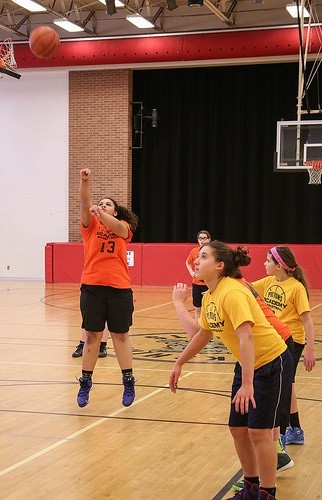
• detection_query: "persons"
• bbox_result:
[169,242,293,500]
[75,168,136,407]
[172,270,293,492]
[186,231,211,325]
[252,246,316,445]
[72,319,109,358]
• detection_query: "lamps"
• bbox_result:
[13,0,47,12]
[286,0,310,18]
[105,0,116,15]
[125,12,155,28]
[188,0,203,8]
[53,18,84,32]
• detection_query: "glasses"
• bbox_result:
[197,237,209,240]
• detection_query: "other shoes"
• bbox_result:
[232,479,245,490]
[276,453,294,473]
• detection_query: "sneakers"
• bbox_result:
[225,480,277,500]
[72,343,84,357]
[121,375,136,407]
[74,375,94,408]
[285,425,305,446]
[98,345,108,357]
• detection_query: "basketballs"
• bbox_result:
[29,26,60,58]
